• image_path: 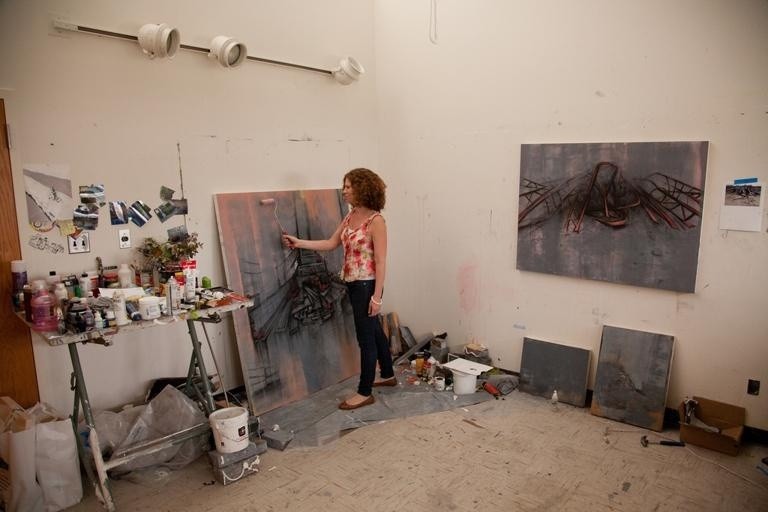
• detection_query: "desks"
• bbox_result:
[11,288,256,511]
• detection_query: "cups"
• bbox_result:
[138,295,161,320]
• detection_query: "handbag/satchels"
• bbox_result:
[0,395,88,512]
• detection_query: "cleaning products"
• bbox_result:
[79,273,92,297]
[47,271,61,292]
[55,283,68,307]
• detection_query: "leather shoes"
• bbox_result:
[373,376,398,387]
[338,393,374,410]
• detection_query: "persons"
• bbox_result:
[280,169,398,411]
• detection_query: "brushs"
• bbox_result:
[260,199,295,250]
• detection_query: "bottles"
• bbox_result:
[111,289,129,326]
[183,265,195,300]
[165,276,181,316]
[10,258,28,313]
[31,268,92,299]
[94,312,103,329]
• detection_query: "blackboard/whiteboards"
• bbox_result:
[212,189,379,416]
[516,142,708,293]
[519,337,591,408]
[590,324,676,432]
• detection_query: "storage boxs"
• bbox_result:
[677,394,746,458]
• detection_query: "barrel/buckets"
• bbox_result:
[449,368,477,395]
[209,406,250,454]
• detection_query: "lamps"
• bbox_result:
[137,22,365,87]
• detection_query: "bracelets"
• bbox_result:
[371,296,383,306]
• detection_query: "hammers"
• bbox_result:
[641,436,685,448]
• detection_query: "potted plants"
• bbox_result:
[135,229,206,286]
